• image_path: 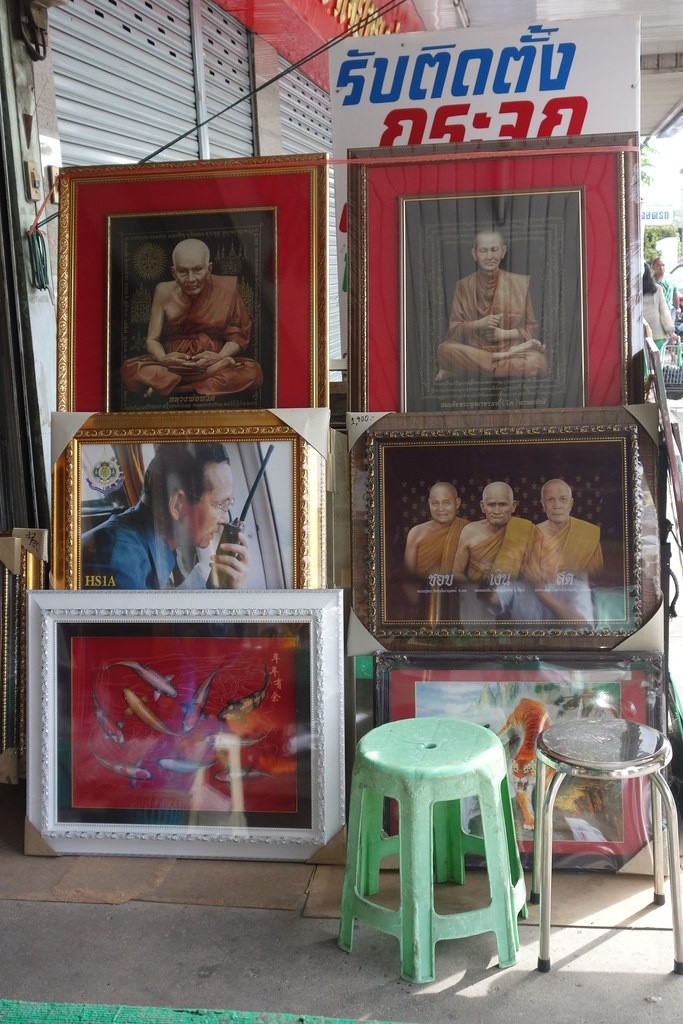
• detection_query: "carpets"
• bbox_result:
[0,1000,399,1023]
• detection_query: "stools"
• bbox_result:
[337,717,529,986]
[531,717,683,974]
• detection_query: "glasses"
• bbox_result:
[172,487,235,514]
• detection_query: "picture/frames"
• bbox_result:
[1,130,683,873]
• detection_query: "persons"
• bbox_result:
[433,229,548,382]
[475,533,596,630]
[452,479,545,585]
[118,239,264,400]
[403,482,475,578]
[82,440,248,590]
[530,479,605,583]
[652,257,680,324]
[643,263,679,380]
